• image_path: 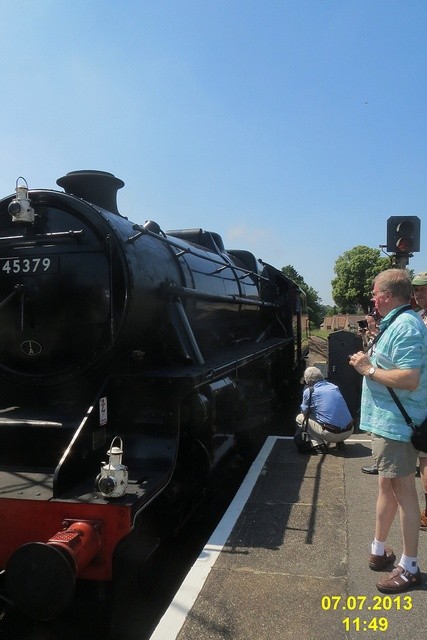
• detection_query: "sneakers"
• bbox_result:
[418,509,427,531]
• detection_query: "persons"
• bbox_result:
[295,367,355,454]
[357,312,379,347]
[350,267,427,593]
[412,272,427,531]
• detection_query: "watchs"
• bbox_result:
[367,366,376,376]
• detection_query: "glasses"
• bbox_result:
[371,289,391,298]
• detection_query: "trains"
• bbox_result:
[0,168,313,620]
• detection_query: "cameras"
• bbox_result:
[357,311,379,328]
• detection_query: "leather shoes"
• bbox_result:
[312,445,330,454]
[336,442,345,449]
[361,466,379,474]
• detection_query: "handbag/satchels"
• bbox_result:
[294,426,315,454]
[411,417,427,454]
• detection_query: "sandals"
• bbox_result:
[369,548,395,571]
[376,565,421,593]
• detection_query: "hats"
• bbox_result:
[411,272,427,286]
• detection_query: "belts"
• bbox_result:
[322,425,353,434]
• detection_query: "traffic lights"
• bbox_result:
[385,216,421,254]
